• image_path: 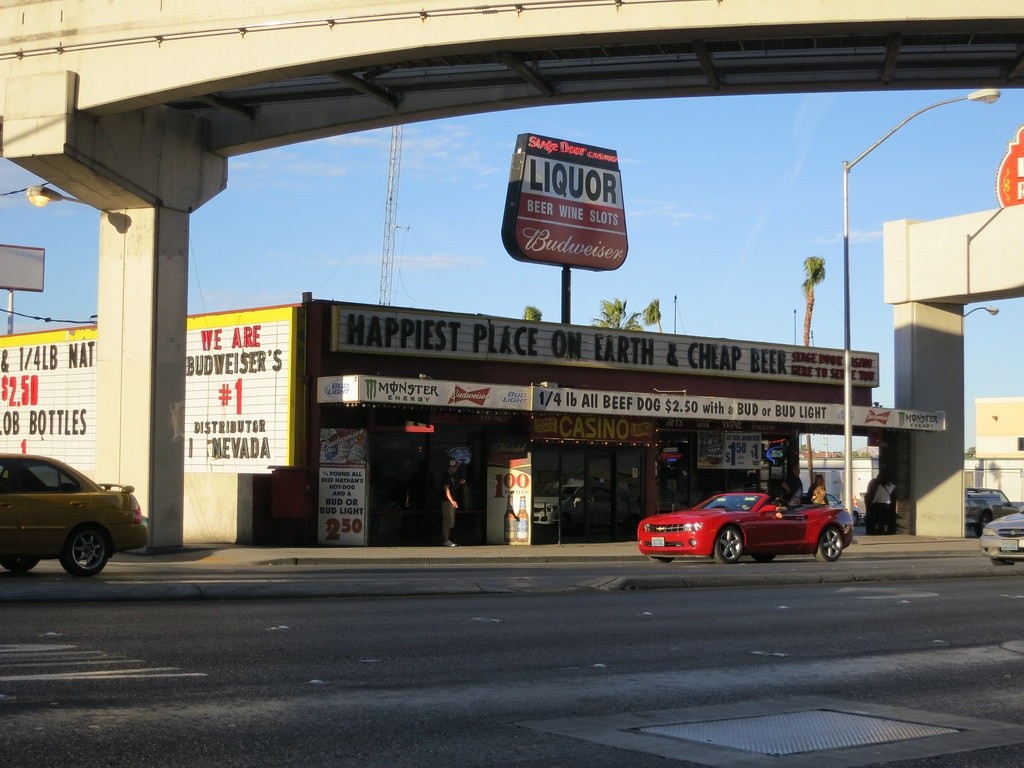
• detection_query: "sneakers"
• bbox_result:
[443,540,457,546]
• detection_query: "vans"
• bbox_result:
[532,476,629,531]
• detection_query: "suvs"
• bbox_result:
[966,488,1020,516]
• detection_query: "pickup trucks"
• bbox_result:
[965,494,1001,535]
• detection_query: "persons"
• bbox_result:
[776,463,803,505]
[865,473,896,535]
[406,462,429,543]
[773,497,790,512]
[808,474,829,506]
[442,462,465,547]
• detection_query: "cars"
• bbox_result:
[803,492,862,525]
[0,454,148,578]
[979,507,1024,565]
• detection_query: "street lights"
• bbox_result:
[843,89,1001,511]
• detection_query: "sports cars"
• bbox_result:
[638,492,853,565]
